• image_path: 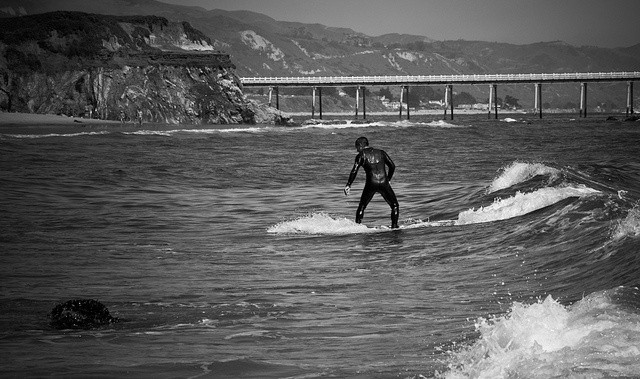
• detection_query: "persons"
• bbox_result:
[344,137,400,228]
